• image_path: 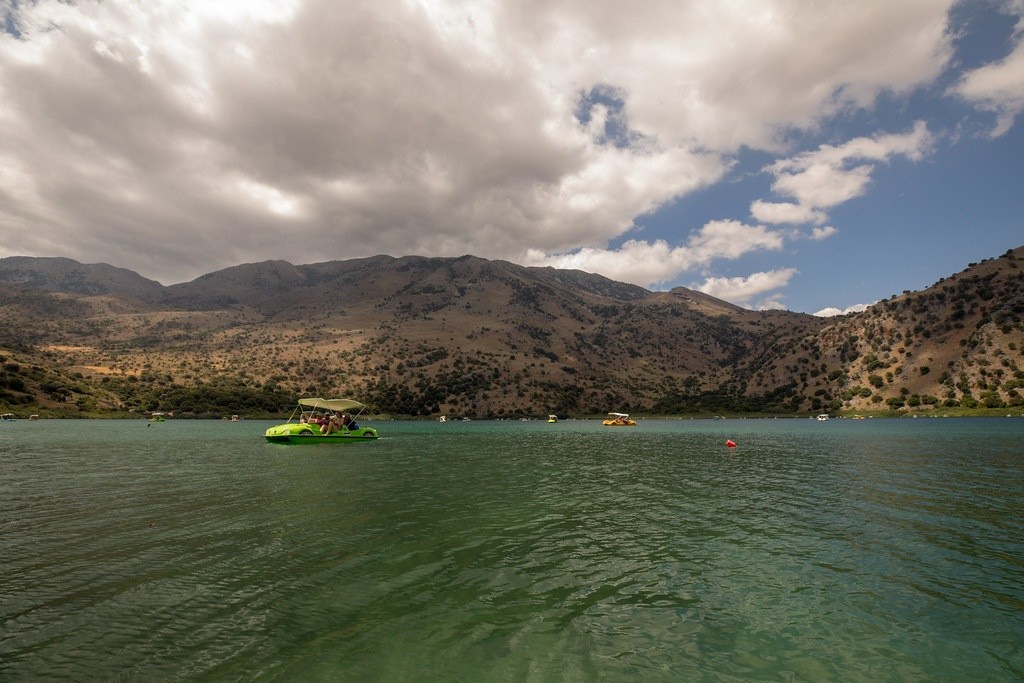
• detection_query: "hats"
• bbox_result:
[316,412,323,419]
[325,412,330,415]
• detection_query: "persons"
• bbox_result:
[311,411,357,436]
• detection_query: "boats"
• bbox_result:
[853,415,865,419]
[147,413,165,421]
[547,414,558,423]
[462,417,470,421]
[29,415,39,421]
[603,412,636,425]
[263,398,379,441]
[0,413,17,422]
[300,411,351,424]
[221,416,227,420]
[817,414,830,421]
[232,415,239,422]
[436,416,451,423]
